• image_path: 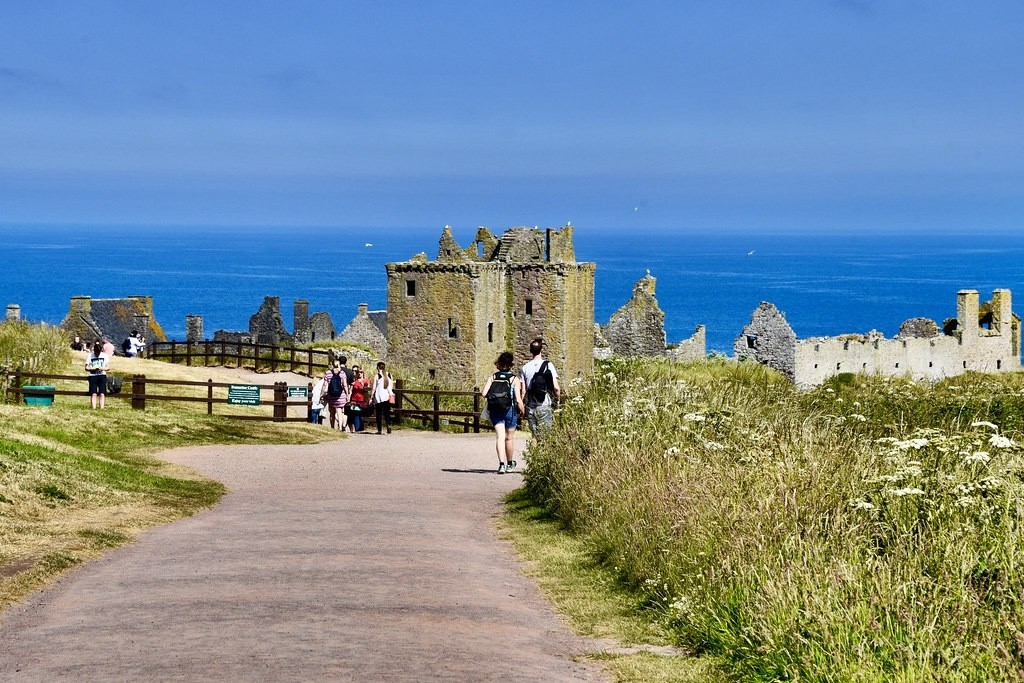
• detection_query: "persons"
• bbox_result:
[68,336,114,356]
[482,352,524,474]
[85,344,110,410]
[369,362,393,434]
[520,341,560,438]
[312,356,371,433]
[125,330,145,357]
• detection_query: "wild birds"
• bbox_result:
[365,243,373,247]
[535,226,537,229]
[646,268,650,274]
[421,252,424,254]
[633,208,637,212]
[745,249,758,256]
[445,225,449,228]
[567,220,571,226]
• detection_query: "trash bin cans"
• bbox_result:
[22,385,56,406]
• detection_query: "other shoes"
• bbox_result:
[375,432,381,434]
[387,426,391,434]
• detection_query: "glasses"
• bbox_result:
[352,370,357,372]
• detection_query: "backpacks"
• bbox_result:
[328,370,343,398]
[521,361,552,405]
[485,372,514,413]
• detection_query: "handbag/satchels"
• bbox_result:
[388,393,396,405]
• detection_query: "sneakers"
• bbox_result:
[497,464,505,473]
[505,461,518,472]
